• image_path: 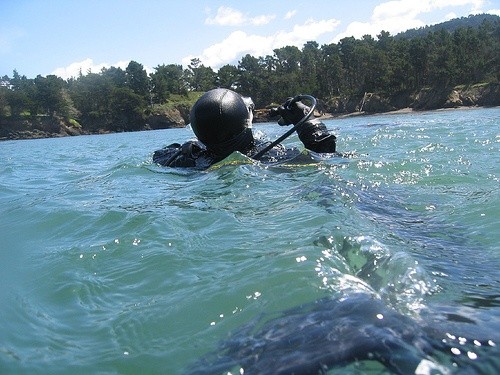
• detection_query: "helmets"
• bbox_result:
[191,89,255,150]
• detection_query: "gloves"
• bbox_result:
[279,96,337,149]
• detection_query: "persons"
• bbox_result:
[154,87,337,174]
